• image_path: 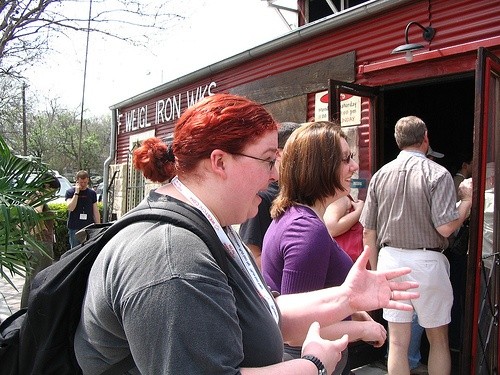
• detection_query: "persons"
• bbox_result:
[0,95,495,375]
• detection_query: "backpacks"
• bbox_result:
[0,206,230,375]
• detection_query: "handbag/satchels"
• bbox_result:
[332,194,372,270]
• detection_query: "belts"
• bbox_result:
[380,242,446,254]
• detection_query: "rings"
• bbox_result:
[391,291,394,300]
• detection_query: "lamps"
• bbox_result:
[392,21,436,62]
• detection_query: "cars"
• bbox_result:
[24,170,74,206]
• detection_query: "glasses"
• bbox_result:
[228,151,277,171]
[342,152,355,162]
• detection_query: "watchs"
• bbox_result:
[302,355,328,375]
[301,355,327,375]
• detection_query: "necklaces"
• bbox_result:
[457,172,466,179]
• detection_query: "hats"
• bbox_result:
[427,145,444,158]
